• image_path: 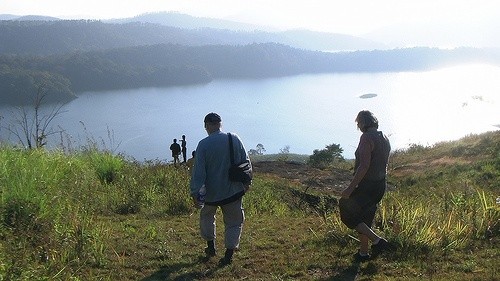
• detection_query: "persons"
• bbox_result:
[341,111,391,260]
[170,138,181,164]
[191,113,252,264]
[179,135,186,162]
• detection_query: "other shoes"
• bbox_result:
[352,249,370,261]
[224,249,234,259]
[205,247,217,256]
[370,237,388,259]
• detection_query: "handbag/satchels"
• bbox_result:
[226,132,253,183]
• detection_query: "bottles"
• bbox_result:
[197,184,206,208]
